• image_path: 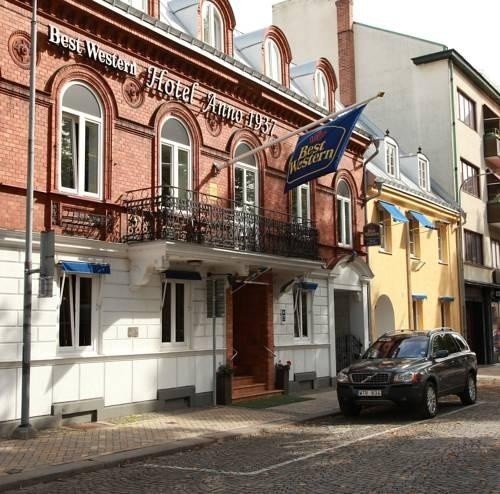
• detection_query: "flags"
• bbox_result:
[283,104,367,193]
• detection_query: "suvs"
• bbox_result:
[337,327,478,418]
